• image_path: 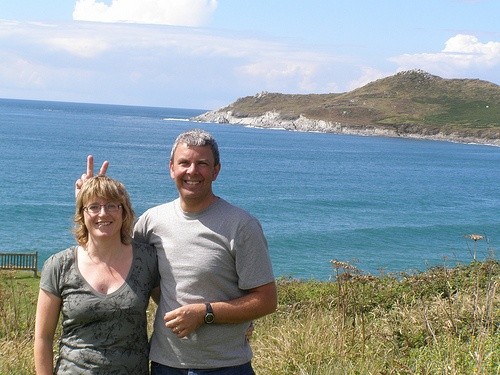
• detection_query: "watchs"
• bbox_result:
[204,302,215,325]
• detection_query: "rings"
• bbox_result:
[175,327,181,334]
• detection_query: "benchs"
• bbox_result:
[0,251,38,277]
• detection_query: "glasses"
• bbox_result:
[81,202,123,213]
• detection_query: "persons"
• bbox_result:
[34,176,255,375]
[74,128,277,375]
